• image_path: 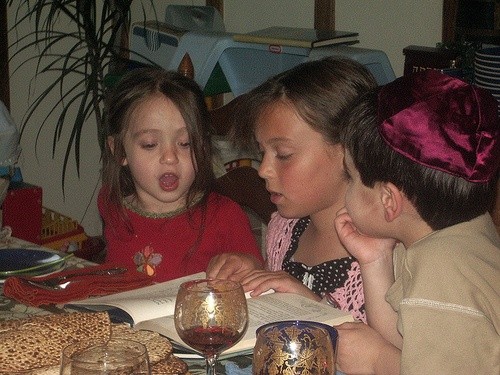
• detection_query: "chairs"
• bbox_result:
[212,166,279,261]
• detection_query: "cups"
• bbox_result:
[61,338,151,375]
[252,322,335,375]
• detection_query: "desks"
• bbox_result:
[1,232,341,375]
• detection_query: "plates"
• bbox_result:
[0,249,68,283]
[474,47,500,118]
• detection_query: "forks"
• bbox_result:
[28,276,126,292]
[13,267,128,286]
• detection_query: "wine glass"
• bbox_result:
[173,280,248,375]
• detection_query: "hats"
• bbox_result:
[374,70,499,183]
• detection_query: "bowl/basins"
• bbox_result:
[257,320,337,375]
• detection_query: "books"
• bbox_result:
[233,26,360,48]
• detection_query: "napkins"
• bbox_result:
[5,264,153,307]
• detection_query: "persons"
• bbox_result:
[208,53,379,327]
[98,65,264,282]
[335,69,500,375]
